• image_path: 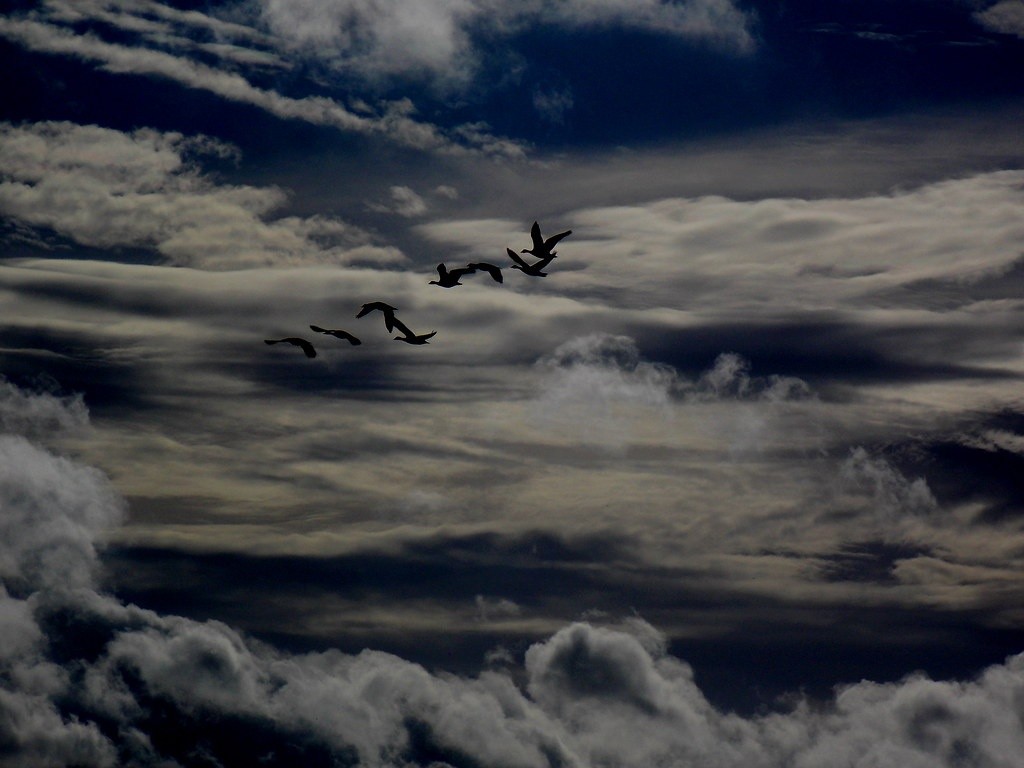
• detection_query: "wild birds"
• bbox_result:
[264,221,573,360]
